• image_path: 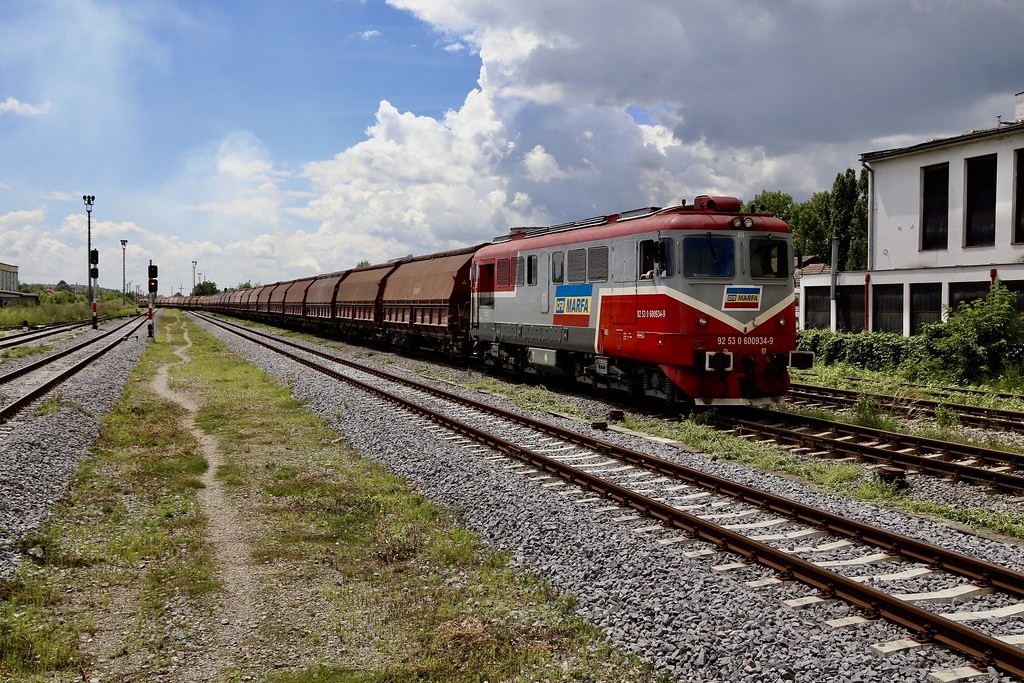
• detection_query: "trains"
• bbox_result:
[136,193,816,418]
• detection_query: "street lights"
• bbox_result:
[192,260,197,287]
[197,273,201,283]
[82,195,96,306]
[119,239,129,304]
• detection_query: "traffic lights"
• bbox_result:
[148,265,158,293]
[90,250,99,279]
